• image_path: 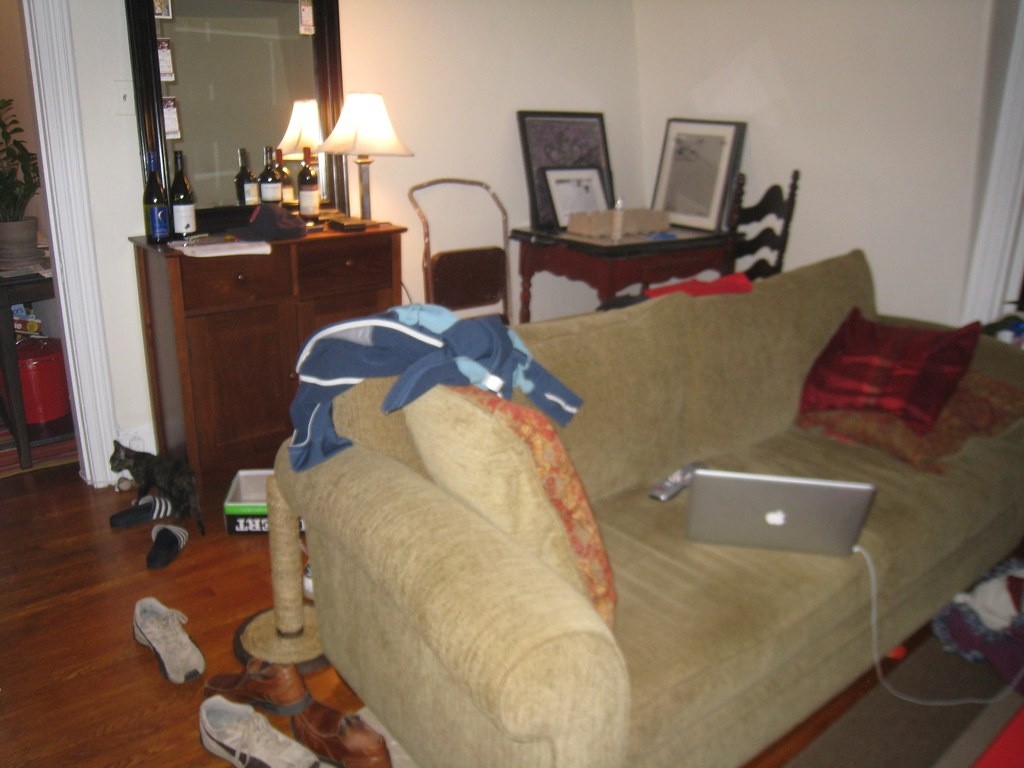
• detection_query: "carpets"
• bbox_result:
[0,412,79,479]
[783,633,1024,768]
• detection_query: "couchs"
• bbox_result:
[274,247,1024,768]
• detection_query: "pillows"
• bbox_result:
[401,381,617,631]
[794,366,1024,479]
[797,300,982,439]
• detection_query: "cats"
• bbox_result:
[109,440,206,537]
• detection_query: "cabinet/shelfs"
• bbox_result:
[124,213,408,514]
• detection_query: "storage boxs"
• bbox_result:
[566,205,669,235]
[221,469,306,535]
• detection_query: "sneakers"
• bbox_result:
[133,596,205,685]
[198,694,319,768]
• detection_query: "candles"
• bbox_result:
[611,197,625,241]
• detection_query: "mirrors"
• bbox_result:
[123,0,351,233]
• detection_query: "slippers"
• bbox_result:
[109,495,175,528]
[146,523,188,568]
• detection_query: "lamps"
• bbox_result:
[278,99,327,200]
[313,92,418,215]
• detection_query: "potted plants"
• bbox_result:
[0,99,45,263]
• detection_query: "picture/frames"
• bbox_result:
[539,164,610,235]
[518,109,615,231]
[654,117,747,235]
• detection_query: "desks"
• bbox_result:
[510,222,747,325]
[0,246,76,470]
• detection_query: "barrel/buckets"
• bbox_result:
[0,338,69,424]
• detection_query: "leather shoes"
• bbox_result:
[204,658,312,715]
[291,701,392,768]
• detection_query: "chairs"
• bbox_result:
[721,169,801,281]
[407,178,511,325]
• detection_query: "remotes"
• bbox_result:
[649,461,704,502]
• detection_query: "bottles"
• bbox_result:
[171,150,198,239]
[297,147,320,224]
[142,152,171,246]
[234,147,256,206]
[257,146,282,205]
[274,149,294,202]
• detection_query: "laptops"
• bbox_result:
[685,467,876,557]
